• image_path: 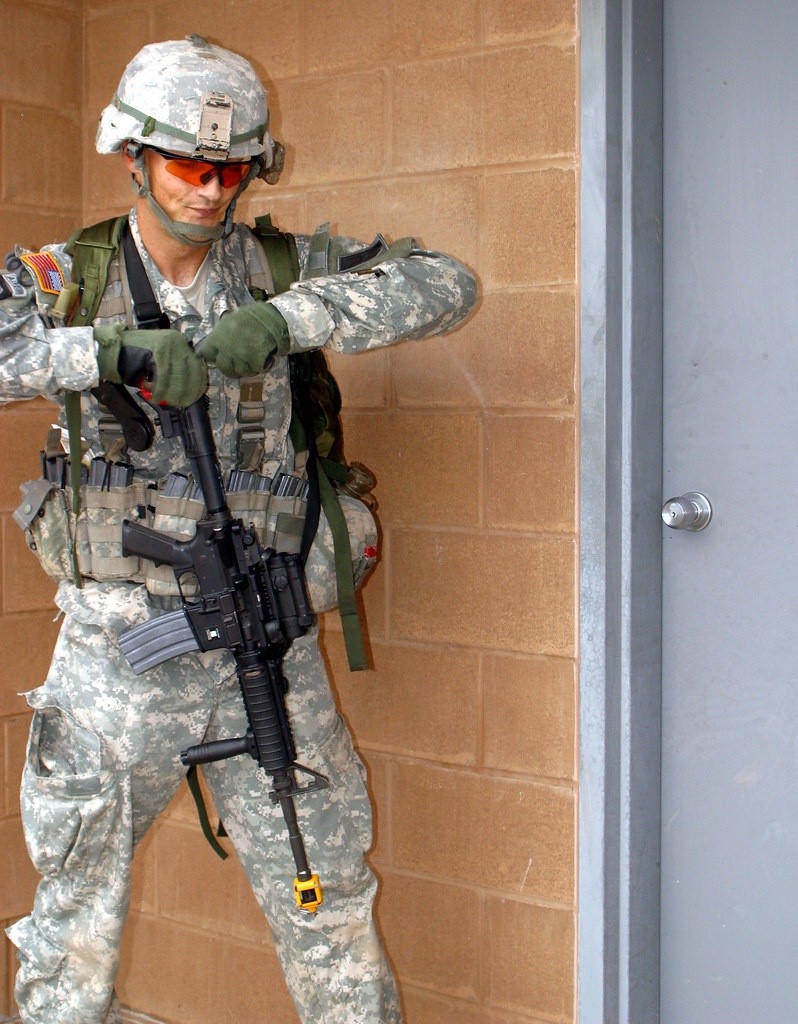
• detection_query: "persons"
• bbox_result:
[0,35,478,1024]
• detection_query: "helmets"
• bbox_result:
[95,33,285,185]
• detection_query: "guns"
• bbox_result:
[118,387,329,913]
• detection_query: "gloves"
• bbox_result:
[97,324,209,407]
[201,301,290,378]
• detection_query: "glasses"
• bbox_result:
[139,143,259,188]
